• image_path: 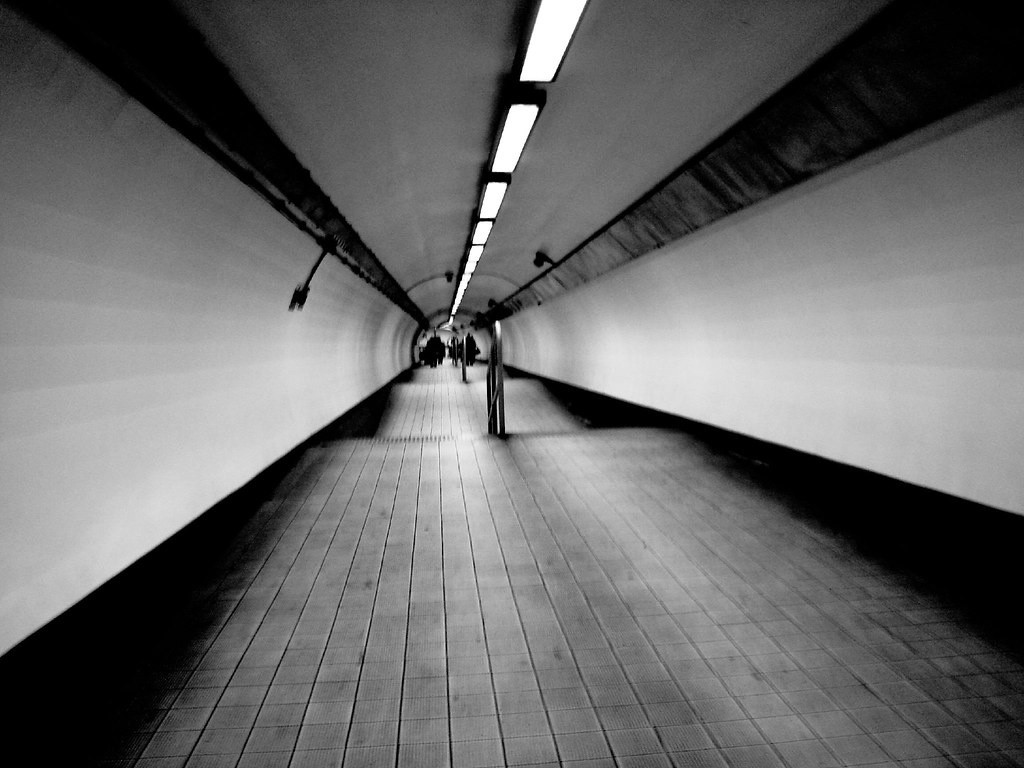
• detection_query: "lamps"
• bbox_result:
[449,0,591,327]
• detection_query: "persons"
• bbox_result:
[447,333,480,366]
[419,332,446,368]
